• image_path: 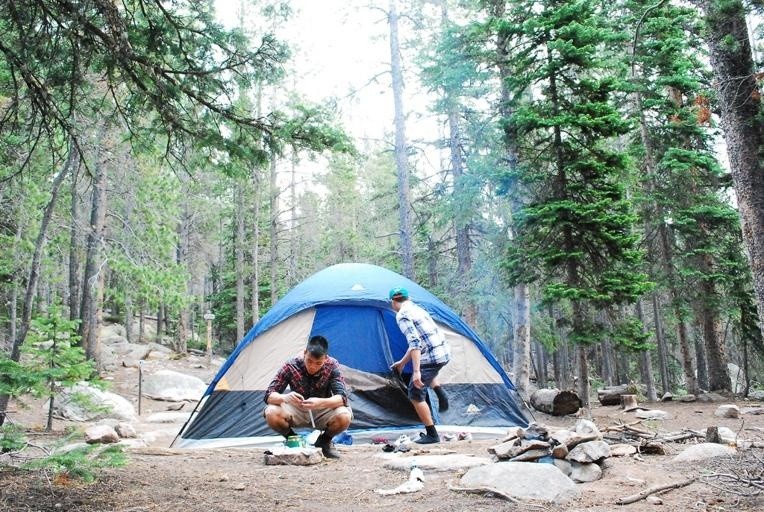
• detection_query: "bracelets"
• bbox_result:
[284,396,290,404]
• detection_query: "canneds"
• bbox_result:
[288,436,303,448]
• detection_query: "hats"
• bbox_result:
[390,287,408,300]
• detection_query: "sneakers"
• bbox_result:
[394,432,472,445]
[314,439,339,458]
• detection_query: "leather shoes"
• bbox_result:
[439,391,448,412]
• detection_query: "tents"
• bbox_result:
[182,262,535,441]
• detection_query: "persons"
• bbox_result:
[263,336,353,460]
[390,284,449,444]
